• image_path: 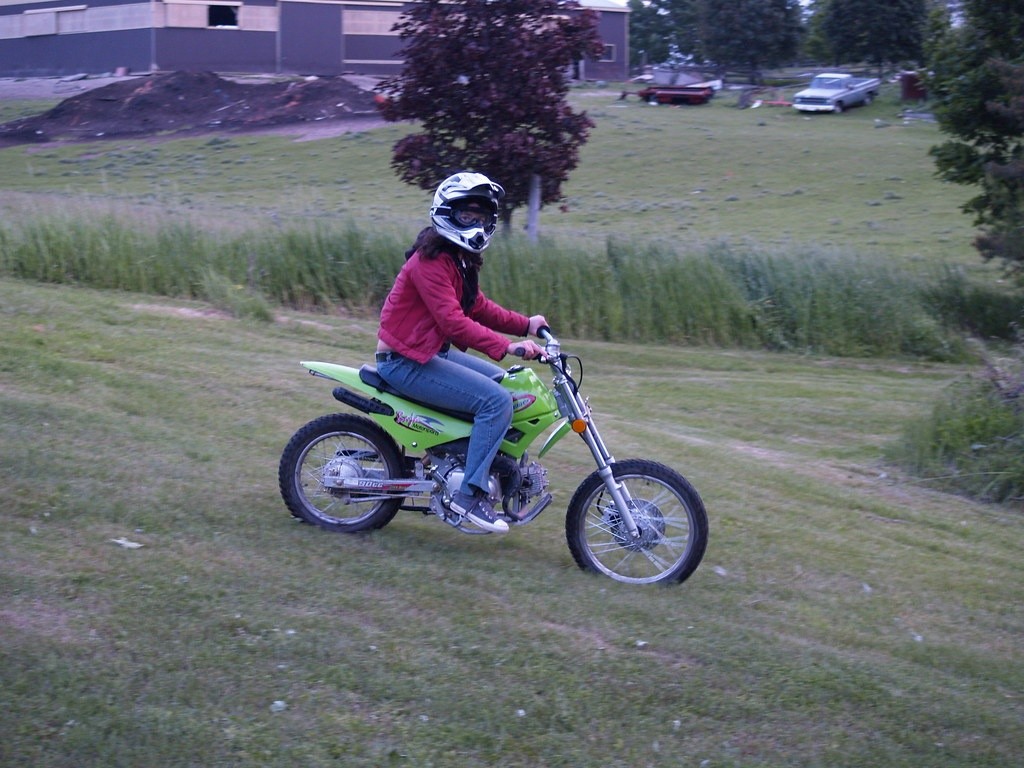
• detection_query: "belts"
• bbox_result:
[374,351,403,361]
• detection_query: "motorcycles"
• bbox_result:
[277,323,710,590]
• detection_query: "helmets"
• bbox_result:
[430,172,506,253]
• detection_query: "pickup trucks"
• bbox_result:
[792,72,881,115]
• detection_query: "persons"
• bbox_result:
[375,172,549,534]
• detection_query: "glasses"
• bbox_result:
[431,205,495,231]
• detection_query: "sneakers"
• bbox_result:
[450,491,510,534]
